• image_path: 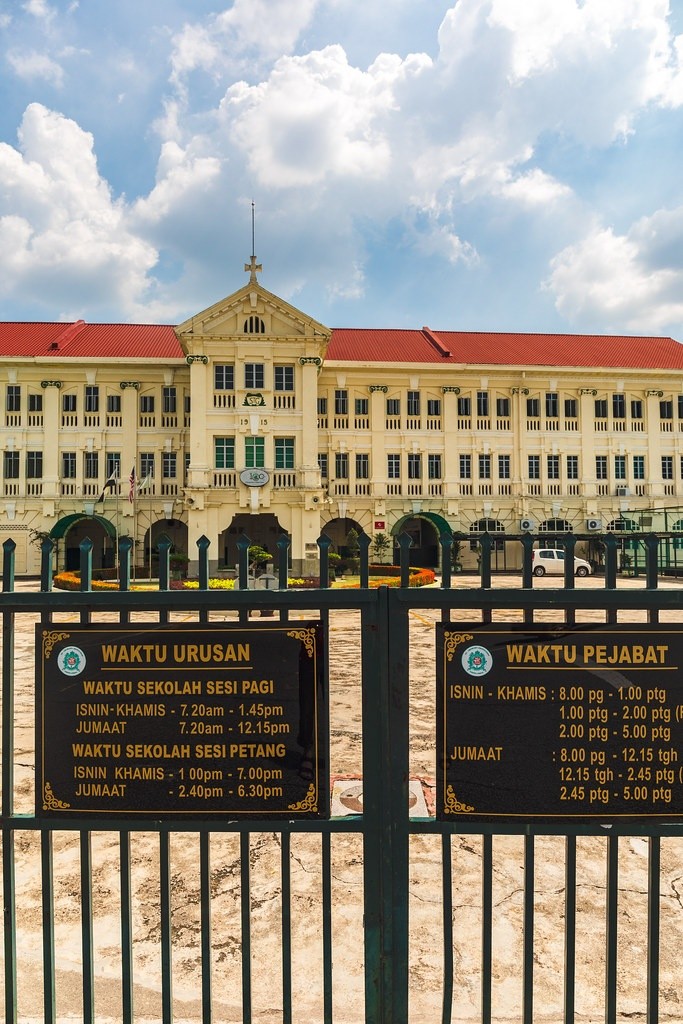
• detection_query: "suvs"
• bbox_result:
[531,548,592,577]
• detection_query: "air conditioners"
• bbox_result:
[520,519,534,531]
[587,519,602,531]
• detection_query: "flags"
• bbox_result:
[97,468,116,503]
[136,473,151,489]
[129,467,134,503]
